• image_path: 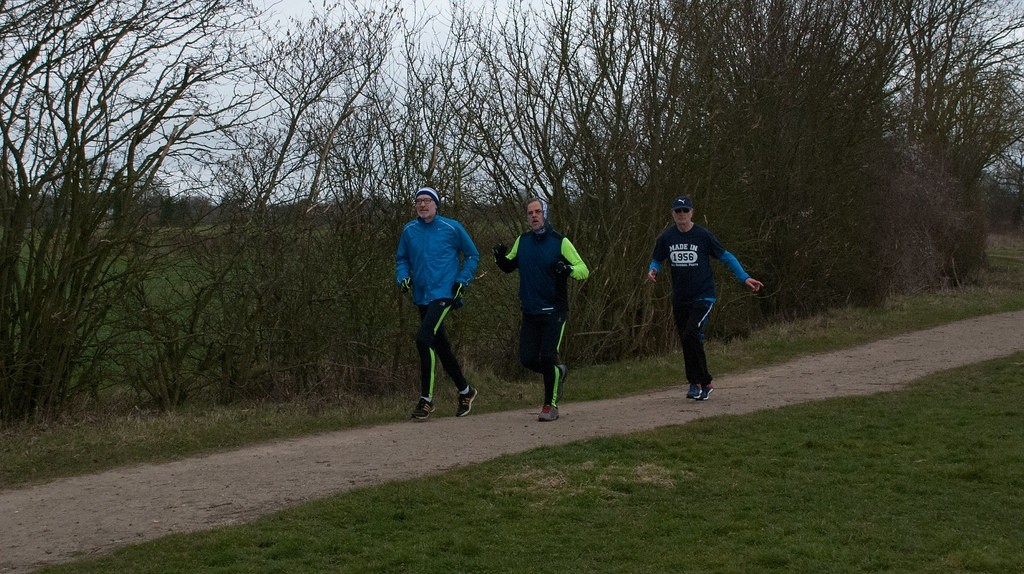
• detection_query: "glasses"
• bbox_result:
[674,208,690,213]
[416,198,434,204]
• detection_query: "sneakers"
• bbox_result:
[555,364,568,403]
[539,404,559,421]
[456,386,478,416]
[411,399,436,420]
[686,384,714,401]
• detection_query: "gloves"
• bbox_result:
[452,283,465,301]
[492,242,507,262]
[554,260,573,275]
[400,277,412,292]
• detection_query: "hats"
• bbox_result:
[416,187,440,206]
[672,196,693,210]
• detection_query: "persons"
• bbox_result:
[647,196,764,401]
[396,187,480,419]
[491,198,589,421]
